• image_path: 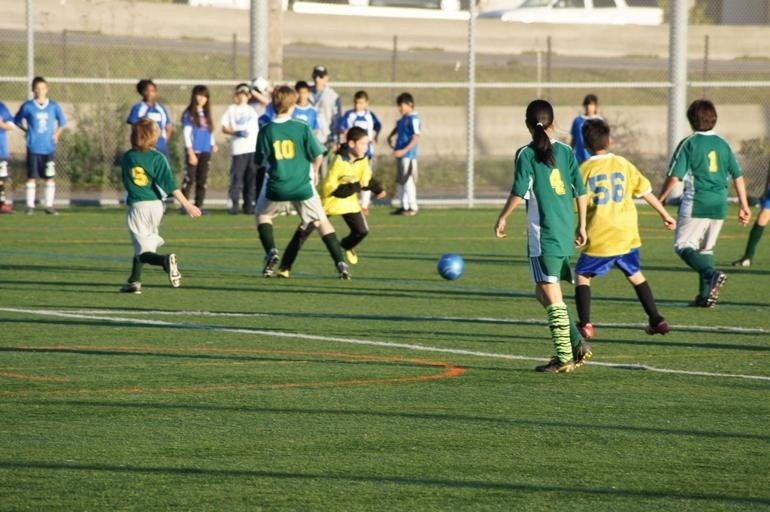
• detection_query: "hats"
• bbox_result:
[312,65,327,78]
[235,84,250,95]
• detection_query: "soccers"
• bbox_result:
[438,251,464,282]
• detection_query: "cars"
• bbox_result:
[477,0,665,25]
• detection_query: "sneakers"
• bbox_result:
[44,206,58,215]
[705,270,728,308]
[345,249,358,266]
[262,249,279,278]
[688,295,706,307]
[535,356,575,374]
[401,209,417,216]
[644,318,669,335]
[389,207,405,215]
[24,206,34,216]
[337,261,351,281]
[574,322,595,340]
[164,253,181,288]
[120,281,142,295]
[733,257,752,267]
[572,342,591,368]
[277,266,289,279]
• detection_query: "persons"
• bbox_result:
[181,85,218,217]
[119,116,202,294]
[259,86,274,125]
[254,86,351,281]
[494,99,593,375]
[289,81,317,135]
[572,118,677,341]
[250,76,271,115]
[126,80,171,162]
[309,66,341,178]
[343,91,386,199]
[0,102,14,214]
[732,156,770,268]
[658,99,750,309]
[387,93,422,218]
[221,84,259,215]
[572,94,603,168]
[276,127,386,278]
[13,76,66,217]
